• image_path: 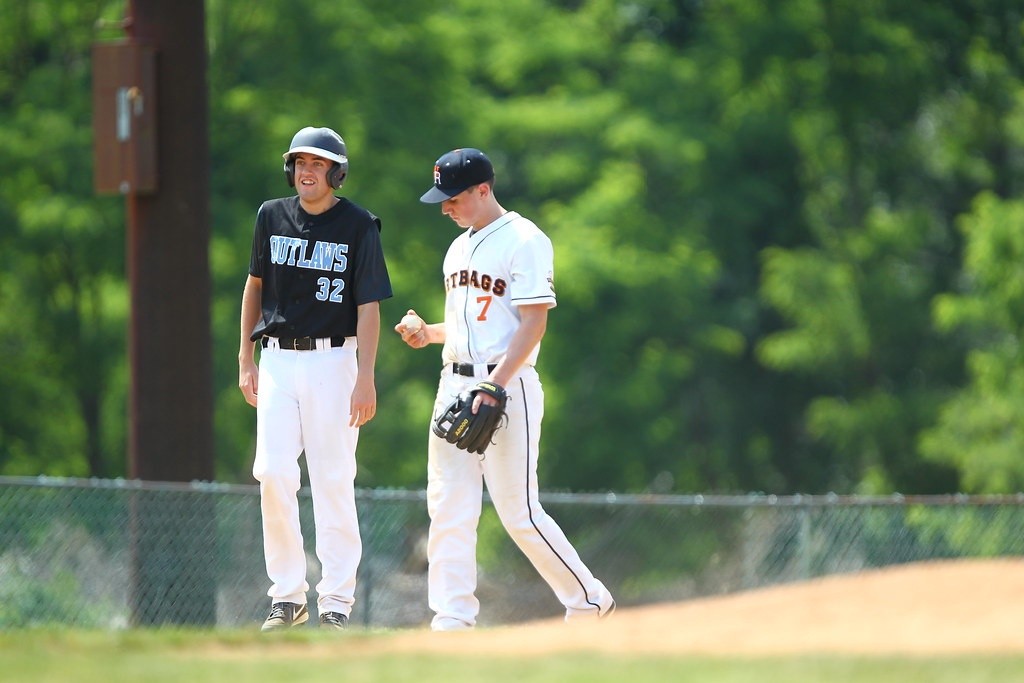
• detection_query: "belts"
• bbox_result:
[453,363,498,377]
[262,336,345,350]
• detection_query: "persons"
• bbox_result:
[394,147,615,633]
[239,126,394,633]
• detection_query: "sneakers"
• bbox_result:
[599,596,616,619]
[317,611,349,632]
[261,602,310,631]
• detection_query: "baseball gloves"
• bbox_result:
[434,383,509,456]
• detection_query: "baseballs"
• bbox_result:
[401,314,422,335]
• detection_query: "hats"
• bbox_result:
[282,126,348,190]
[420,148,495,204]
